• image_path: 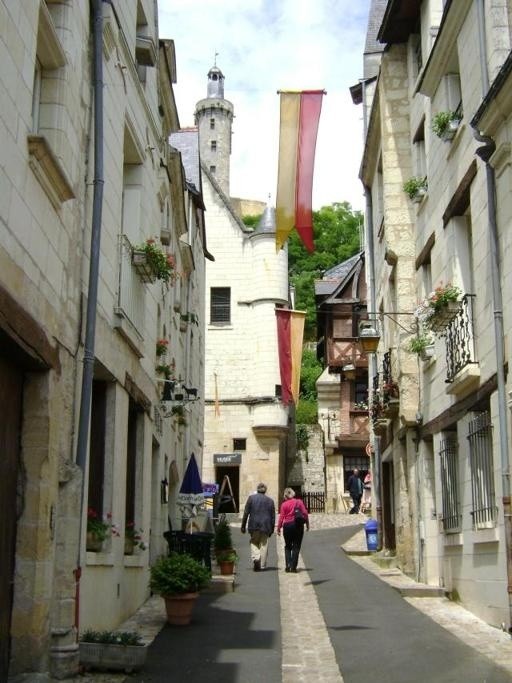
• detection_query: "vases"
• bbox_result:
[132,249,155,284]
[372,418,388,436]
[427,300,462,332]
[125,538,134,554]
[87,531,102,551]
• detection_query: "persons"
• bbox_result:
[240,482,275,572]
[276,487,310,572]
[345,468,365,514]
[362,469,372,509]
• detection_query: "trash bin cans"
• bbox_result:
[364,520,378,551]
[163,531,215,580]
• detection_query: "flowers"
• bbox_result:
[137,239,177,290]
[87,507,120,539]
[354,383,401,423]
[125,518,146,548]
[156,339,170,356]
[414,281,461,323]
[157,363,175,378]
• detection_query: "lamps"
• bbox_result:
[355,313,419,353]
[339,354,367,380]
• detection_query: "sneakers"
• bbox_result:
[253,559,260,572]
[286,566,296,572]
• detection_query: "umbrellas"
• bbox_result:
[176,450,207,536]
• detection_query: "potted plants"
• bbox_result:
[79,630,147,673]
[403,112,462,202]
[149,515,239,625]
[410,333,434,360]
[179,312,199,332]
[178,417,186,432]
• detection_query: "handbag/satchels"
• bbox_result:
[294,507,306,525]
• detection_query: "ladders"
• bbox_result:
[214,474,238,512]
